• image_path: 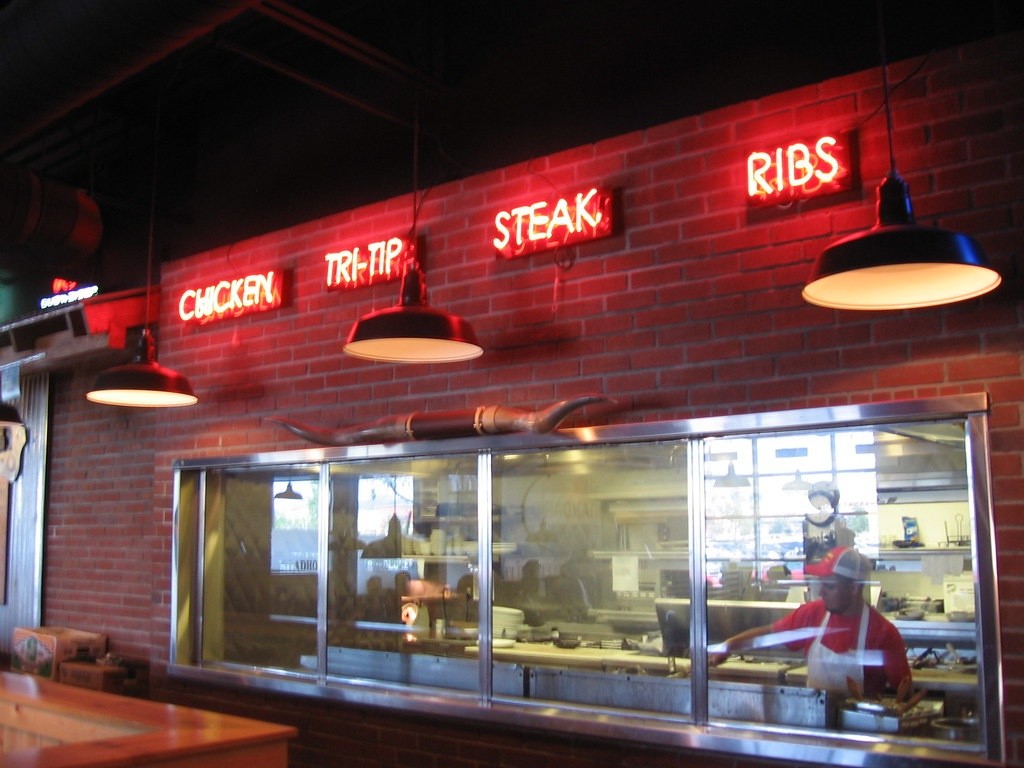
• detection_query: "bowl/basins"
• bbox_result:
[403,529,467,556]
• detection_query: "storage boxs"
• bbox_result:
[10,626,107,683]
[59,661,145,700]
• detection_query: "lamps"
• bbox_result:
[342,184,484,365]
[358,473,423,559]
[801,57,1002,312]
[83,209,200,407]
[274,478,302,500]
[714,458,749,488]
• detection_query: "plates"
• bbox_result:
[556,640,580,648]
[492,542,517,553]
[476,639,516,648]
[492,606,525,640]
[464,541,478,555]
[579,640,623,649]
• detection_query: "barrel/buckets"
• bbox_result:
[930,718,979,743]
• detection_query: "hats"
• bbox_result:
[804,547,871,580]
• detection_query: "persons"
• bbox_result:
[706,546,912,705]
